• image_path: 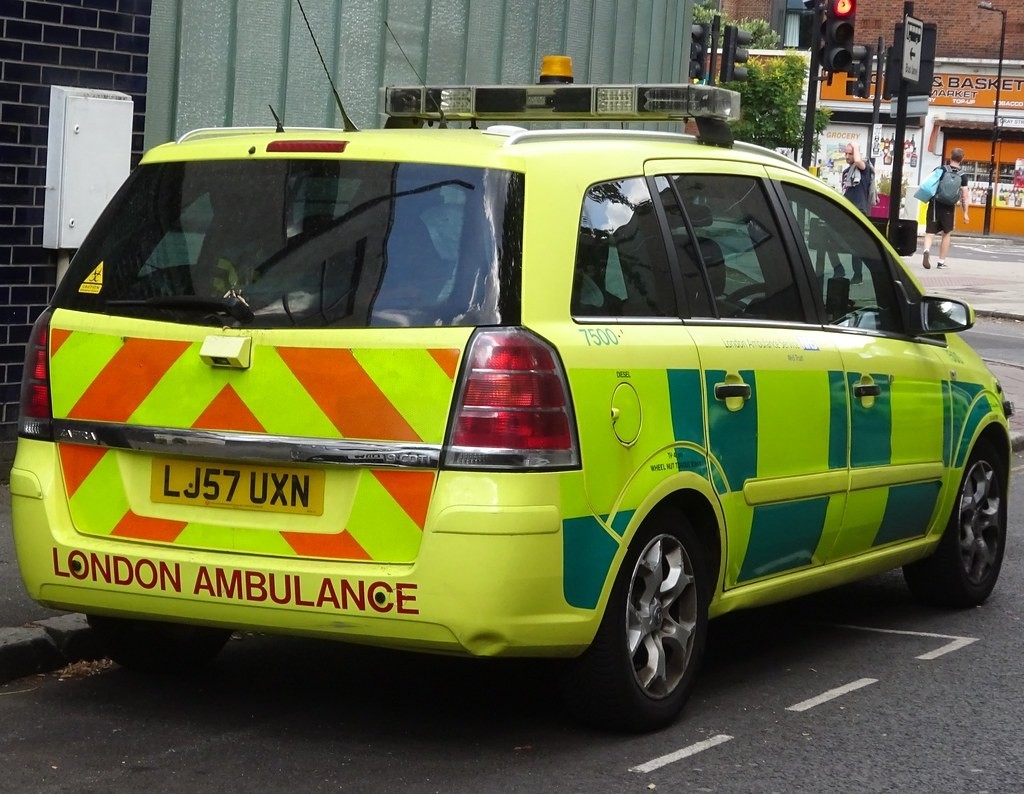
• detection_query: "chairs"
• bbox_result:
[638,209,726,318]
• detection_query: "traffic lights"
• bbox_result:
[718,26,754,82]
[689,22,711,80]
[845,45,875,100]
[821,0,858,75]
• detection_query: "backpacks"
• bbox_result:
[933,164,966,205]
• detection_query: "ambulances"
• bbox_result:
[8,53,1014,734]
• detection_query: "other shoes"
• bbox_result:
[937,261,948,268]
[850,276,863,285]
[922,249,930,269]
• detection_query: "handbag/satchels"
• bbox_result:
[913,167,943,203]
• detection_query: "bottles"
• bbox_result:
[960,161,989,182]
[999,185,1024,207]
[999,164,1015,184]
[970,183,987,204]
[1018,167,1022,176]
[872,132,895,165]
[904,134,917,167]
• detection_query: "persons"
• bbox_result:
[827,143,872,284]
[923,148,970,268]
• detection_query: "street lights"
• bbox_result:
[976,2,1009,234]
